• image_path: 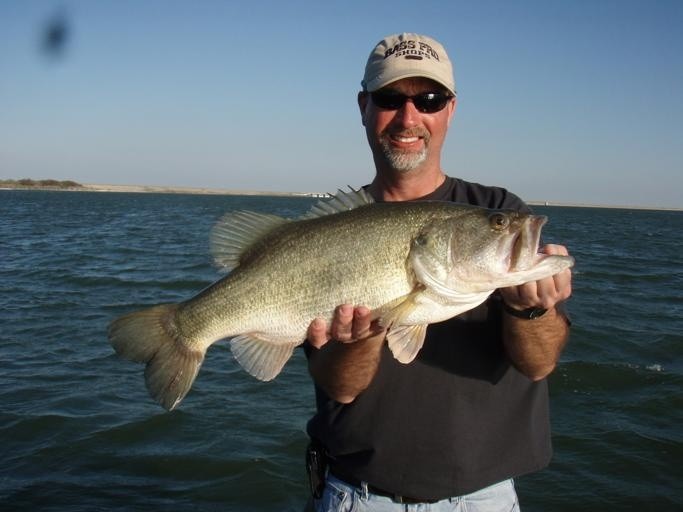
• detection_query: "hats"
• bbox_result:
[360,32,456,98]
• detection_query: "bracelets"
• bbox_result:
[499,296,547,320]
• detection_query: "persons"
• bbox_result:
[304,32,572,512]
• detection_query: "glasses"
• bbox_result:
[370,86,452,114]
